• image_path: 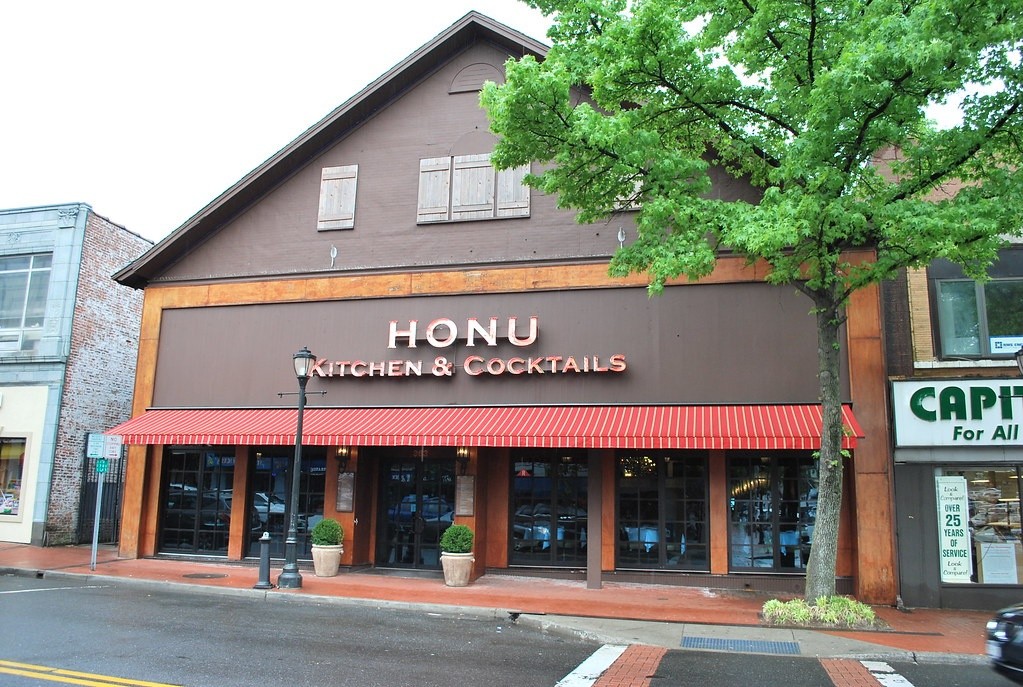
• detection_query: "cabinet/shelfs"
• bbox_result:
[986,498,1021,530]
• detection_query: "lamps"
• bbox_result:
[456,446,471,475]
[335,446,349,474]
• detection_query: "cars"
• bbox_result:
[384,488,829,566]
[162,483,324,549]
[983,602,1023,686]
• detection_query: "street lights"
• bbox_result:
[278,347,329,590]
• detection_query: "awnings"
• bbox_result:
[102,404,866,452]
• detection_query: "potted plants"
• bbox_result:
[439,524,475,587]
[311,518,344,577]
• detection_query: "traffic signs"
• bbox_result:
[87,433,104,459]
[104,435,122,459]
